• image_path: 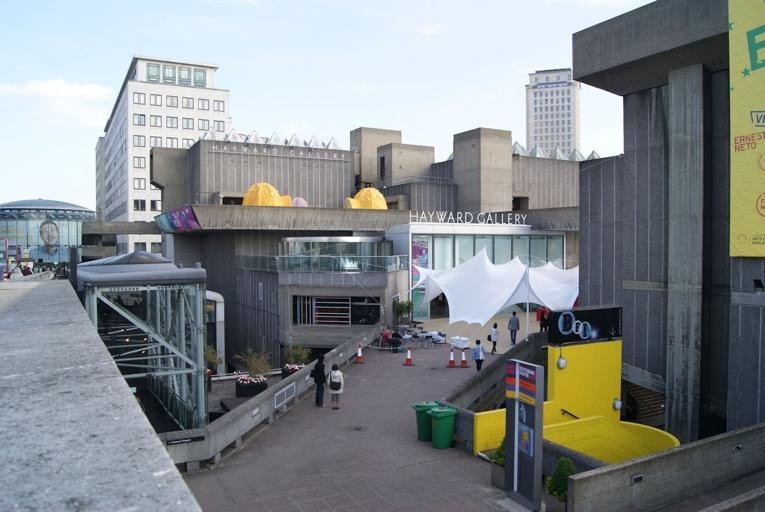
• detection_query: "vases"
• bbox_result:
[282,366,303,379]
[236,380,267,397]
[203,373,212,393]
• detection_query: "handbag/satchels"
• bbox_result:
[479,350,486,361]
[310,369,315,377]
[488,334,491,341]
[330,382,340,390]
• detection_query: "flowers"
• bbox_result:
[282,345,311,371]
[203,344,223,375]
[233,346,275,384]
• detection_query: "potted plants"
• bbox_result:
[544,456,577,512]
[489,436,506,489]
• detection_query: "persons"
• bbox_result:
[18,262,65,275]
[491,323,500,355]
[383,326,392,351]
[540,305,548,332]
[312,354,327,407]
[471,339,486,371]
[390,329,403,353]
[325,364,344,410]
[508,311,520,345]
[28,221,72,261]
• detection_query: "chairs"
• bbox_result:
[380,326,447,353]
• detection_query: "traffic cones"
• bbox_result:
[460,349,469,368]
[447,348,456,368]
[403,349,414,365]
[354,344,365,364]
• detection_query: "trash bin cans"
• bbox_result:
[411,400,459,449]
